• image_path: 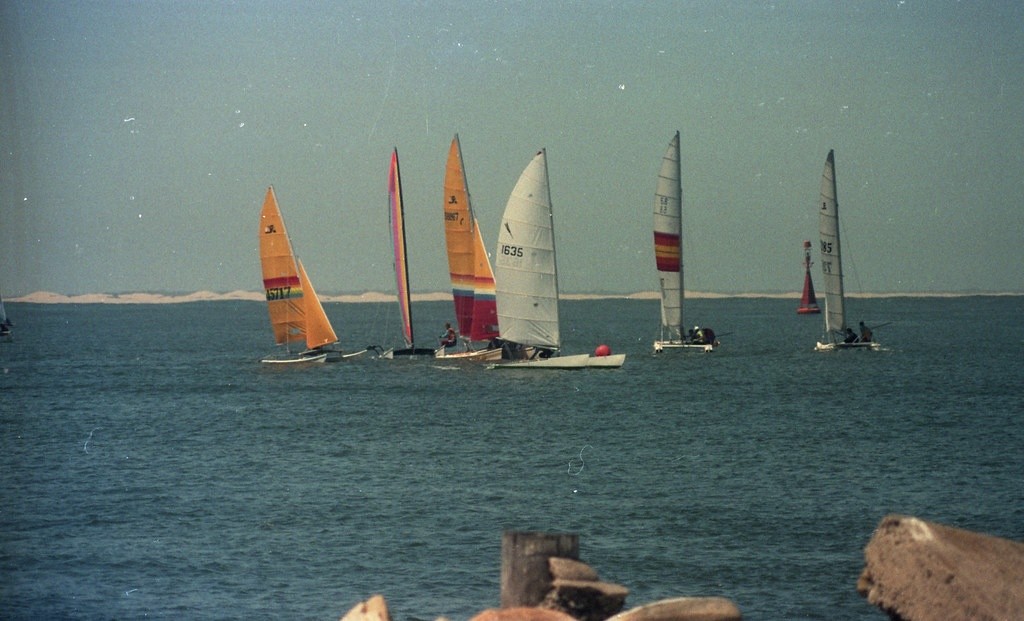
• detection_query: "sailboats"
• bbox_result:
[434,131,543,360]
[488,148,628,369]
[810,149,883,351]
[796,240,822,315]
[650,130,721,352]
[366,147,435,360]
[257,186,368,364]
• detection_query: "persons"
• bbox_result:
[844,328,859,343]
[859,321,872,342]
[439,323,456,347]
[687,326,703,345]
